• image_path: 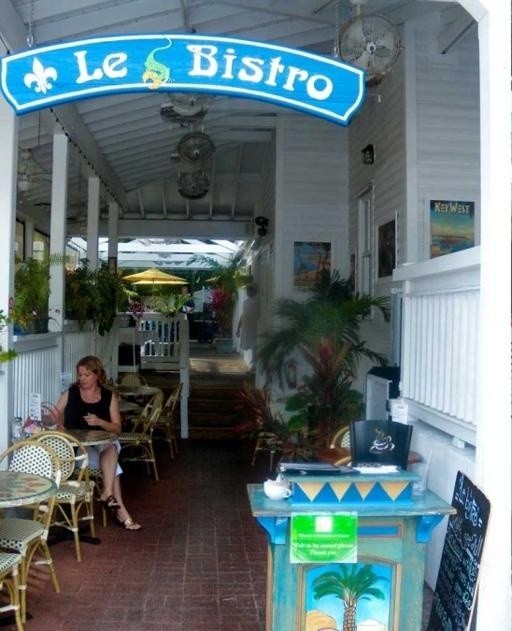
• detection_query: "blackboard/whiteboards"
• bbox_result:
[427,471,490,631]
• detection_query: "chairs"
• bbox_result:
[25,431,106,561]
[115,374,184,481]
[0,443,60,630]
[349,418,414,474]
[329,424,351,450]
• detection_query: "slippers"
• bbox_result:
[98,495,120,509]
[116,516,142,531]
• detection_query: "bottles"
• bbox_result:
[12,416,23,442]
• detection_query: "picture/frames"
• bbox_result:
[346,247,358,297]
[422,193,480,262]
[373,207,402,284]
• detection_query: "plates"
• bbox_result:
[280,461,358,477]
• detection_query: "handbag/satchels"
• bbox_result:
[22,401,64,433]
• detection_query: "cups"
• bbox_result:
[411,462,428,495]
[263,480,293,500]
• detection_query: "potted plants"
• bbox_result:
[13,254,71,336]
[185,254,258,355]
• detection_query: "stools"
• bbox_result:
[251,425,289,474]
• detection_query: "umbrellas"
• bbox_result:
[122,267,187,312]
[130,280,189,285]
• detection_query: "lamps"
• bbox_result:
[18,174,35,194]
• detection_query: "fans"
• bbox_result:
[16,150,53,197]
[334,1,404,87]
[158,90,216,199]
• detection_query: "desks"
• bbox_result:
[316,446,425,465]
[12,430,117,447]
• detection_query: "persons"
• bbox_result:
[24,355,142,531]
[236,282,257,371]
[179,284,194,315]
